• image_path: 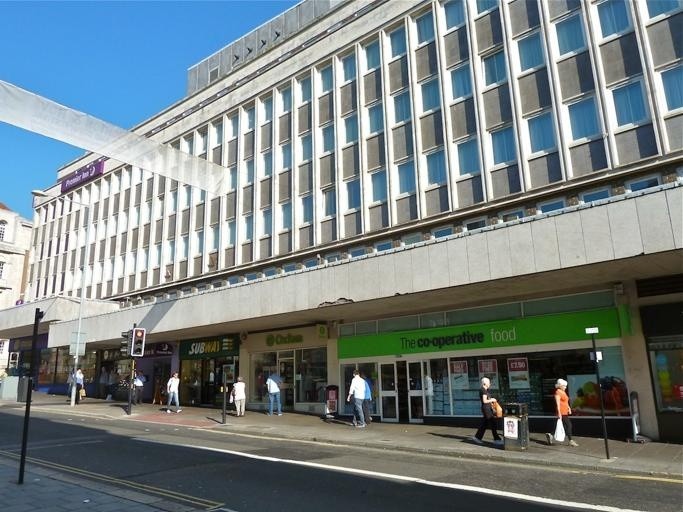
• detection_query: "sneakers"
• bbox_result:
[493,439,504,444]
[166,408,182,414]
[545,433,553,445]
[471,436,482,444]
[349,417,372,428]
[567,439,579,447]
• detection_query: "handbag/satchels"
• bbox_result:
[490,400,503,418]
[78,388,85,400]
[228,391,233,403]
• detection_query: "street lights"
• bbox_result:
[29,189,93,409]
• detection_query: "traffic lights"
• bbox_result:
[120,331,132,357]
[131,328,146,357]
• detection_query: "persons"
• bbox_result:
[38,360,49,383]
[99,366,109,398]
[421,369,433,415]
[133,369,146,405]
[230,376,247,417]
[414,371,422,418]
[104,368,117,400]
[545,377,580,447]
[65,367,74,402]
[165,370,182,413]
[356,372,372,425]
[72,367,84,404]
[266,367,284,416]
[254,361,265,401]
[472,376,504,446]
[223,365,233,380]
[346,369,367,427]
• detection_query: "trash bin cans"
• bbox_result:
[503,403,530,451]
[16,375,28,400]
